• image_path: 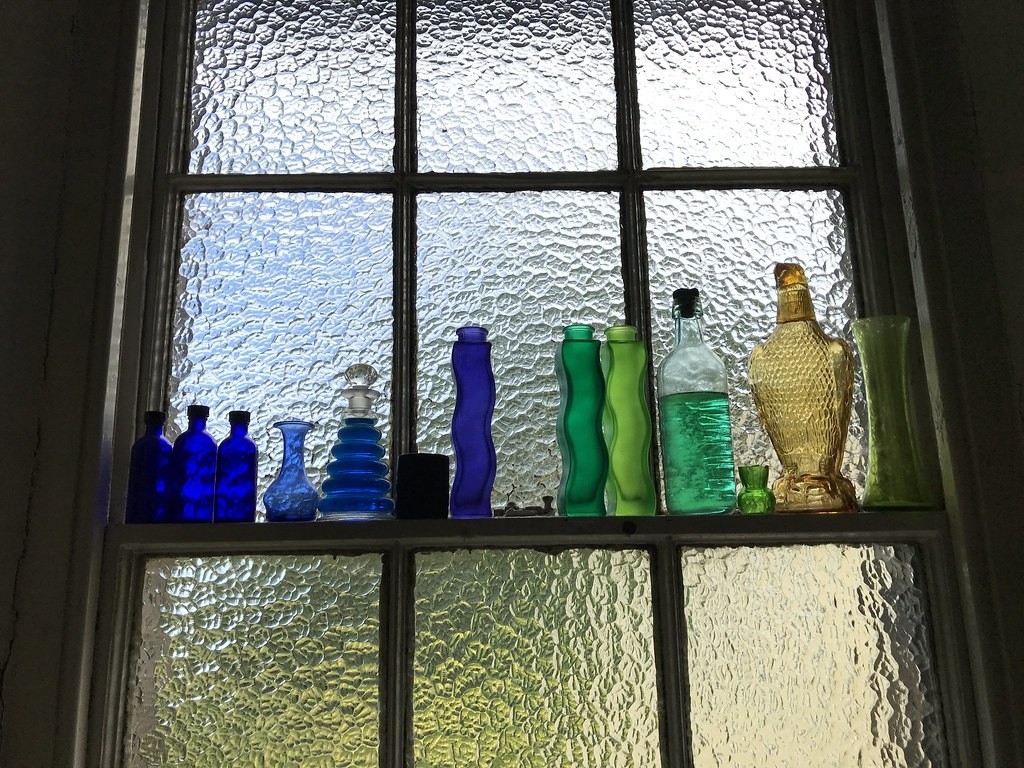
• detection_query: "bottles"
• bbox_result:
[849,314,928,507]
[746,262,856,510]
[601,326,658,516]
[217,410,258,522]
[657,286,737,514]
[125,409,173,523]
[173,404,218,522]
[552,324,607,517]
[262,420,319,520]
[449,326,499,518]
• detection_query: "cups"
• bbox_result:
[393,453,450,522]
